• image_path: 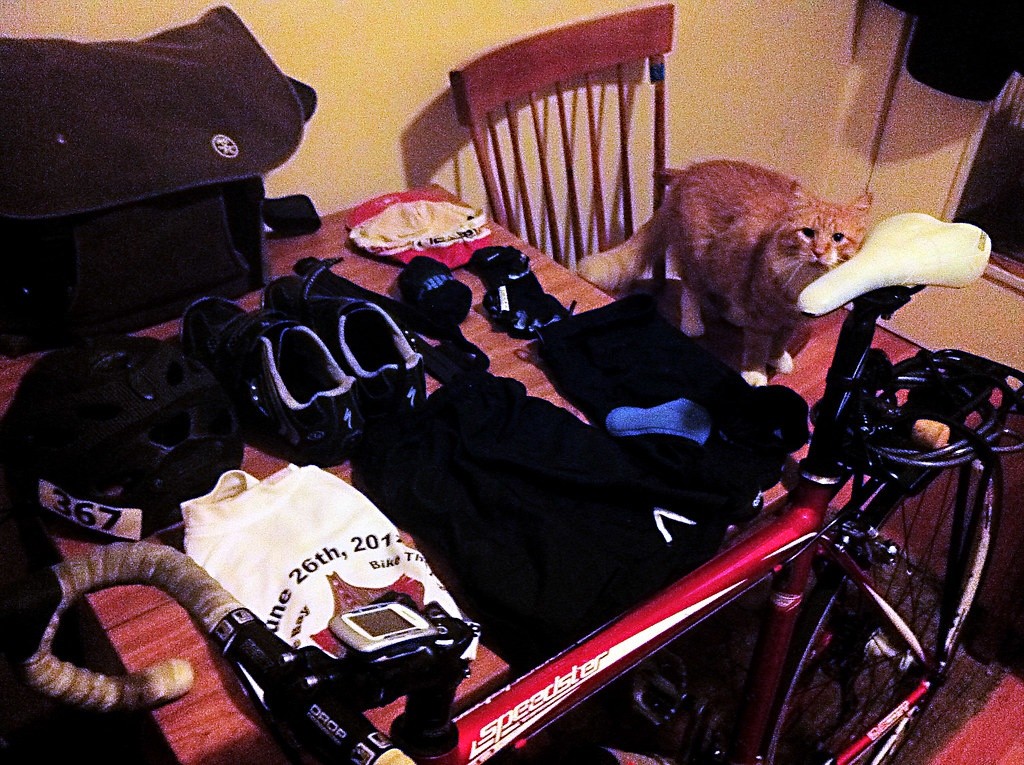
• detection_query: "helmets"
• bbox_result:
[0,335,244,544]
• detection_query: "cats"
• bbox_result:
[574,157,874,390]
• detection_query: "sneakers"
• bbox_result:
[179,275,426,467]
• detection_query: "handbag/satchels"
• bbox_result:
[0,7,316,357]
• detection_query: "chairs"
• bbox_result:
[449,2,811,380]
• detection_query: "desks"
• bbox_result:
[0,185,829,765]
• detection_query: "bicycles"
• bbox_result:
[0,211,1024,765]
